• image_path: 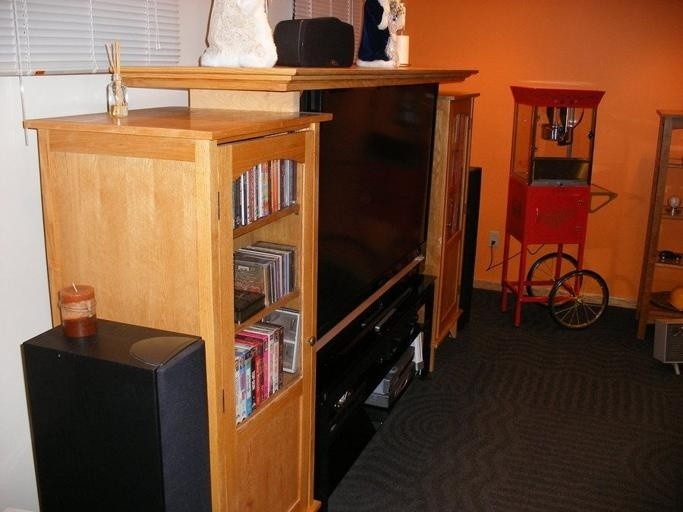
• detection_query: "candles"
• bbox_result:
[58,284,97,339]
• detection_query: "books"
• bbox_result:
[232,157,303,423]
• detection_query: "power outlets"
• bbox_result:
[488,231,497,246]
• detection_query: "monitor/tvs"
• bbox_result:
[298,79,440,352]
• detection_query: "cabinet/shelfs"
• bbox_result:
[23,106,334,512]
[635,110,683,340]
[417,93,482,372]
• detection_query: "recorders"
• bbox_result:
[274,16,354,68]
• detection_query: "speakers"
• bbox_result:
[20,318,212,512]
[460,165,484,328]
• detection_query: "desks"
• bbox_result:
[109,68,479,512]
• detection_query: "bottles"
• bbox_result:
[106,72,130,119]
